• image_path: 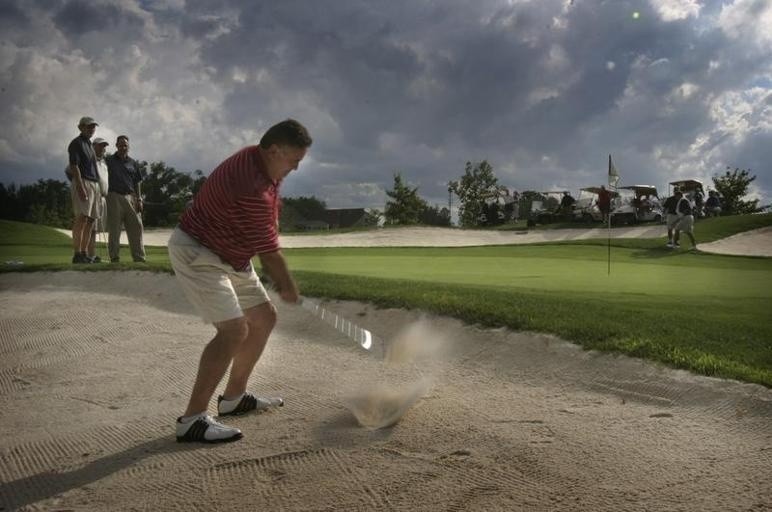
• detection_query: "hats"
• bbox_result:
[80,117,99,126]
[94,138,109,146]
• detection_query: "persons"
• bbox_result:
[479,188,520,224]
[104,135,147,263]
[68,116,100,264]
[64,138,109,263]
[168,117,313,445]
[554,185,630,224]
[639,185,722,255]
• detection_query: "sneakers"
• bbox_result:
[218,390,284,416]
[176,413,244,445]
[73,251,148,265]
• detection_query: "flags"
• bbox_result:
[608,154,620,190]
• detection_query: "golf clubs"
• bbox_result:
[279,291,372,352]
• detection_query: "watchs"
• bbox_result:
[137,198,143,201]
